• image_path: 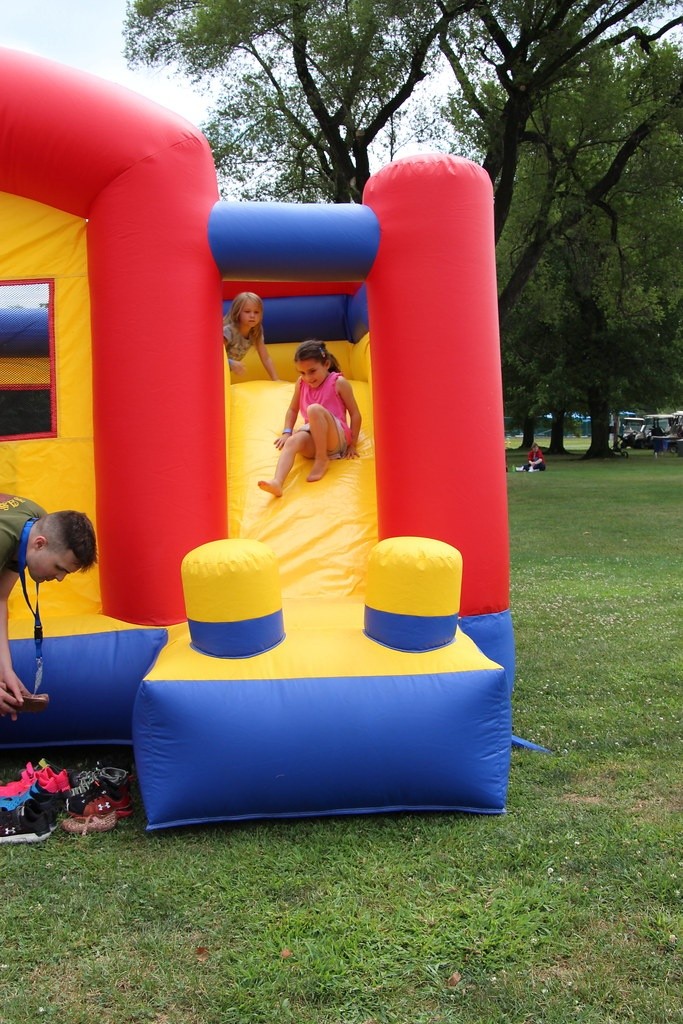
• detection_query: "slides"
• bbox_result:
[133,377,520,830]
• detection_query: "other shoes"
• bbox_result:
[0,758,137,843]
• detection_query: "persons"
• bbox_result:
[522,443,546,472]
[222,292,280,381]
[0,493,97,721]
[257,340,362,497]
[651,422,665,458]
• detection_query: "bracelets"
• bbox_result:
[229,359,233,366]
[282,428,292,435]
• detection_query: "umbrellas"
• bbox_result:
[537,409,636,436]
